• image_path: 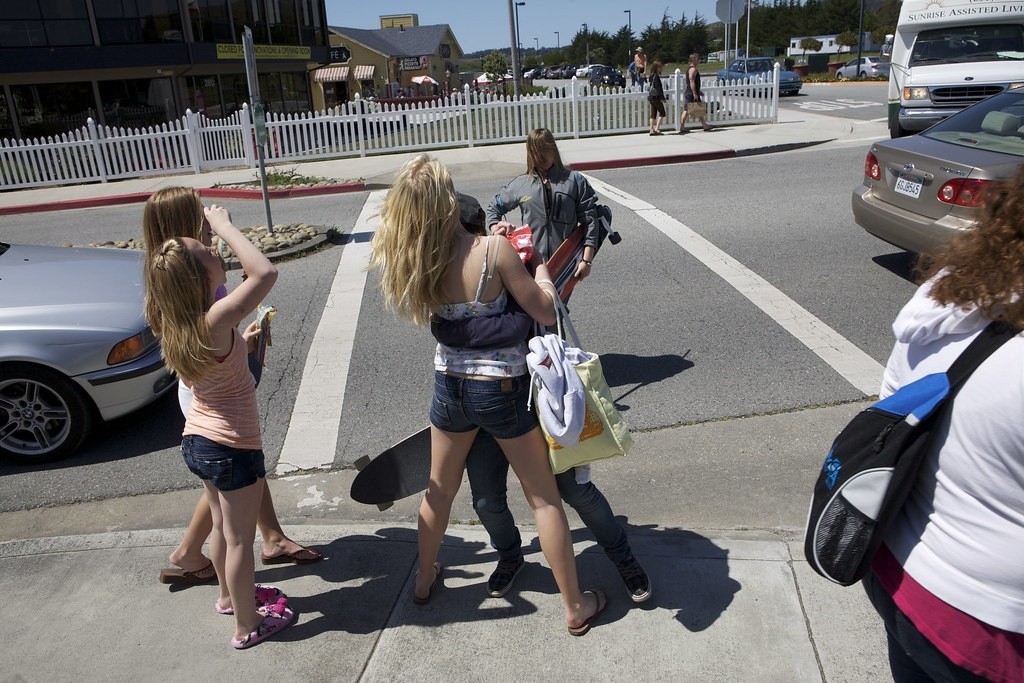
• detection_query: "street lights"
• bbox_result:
[581,23,590,66]
[479,54,484,71]
[534,37,539,51]
[554,32,560,48]
[624,9,632,65]
[519,43,523,60]
[385,57,395,98]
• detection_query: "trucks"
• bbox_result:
[881,0,1024,137]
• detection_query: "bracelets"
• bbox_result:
[582,259,592,266]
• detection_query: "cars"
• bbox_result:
[1,241,182,464]
[835,56,890,80]
[717,56,805,96]
[576,64,605,77]
[590,66,626,89]
[850,84,1024,265]
[520,65,579,79]
[507,70,513,76]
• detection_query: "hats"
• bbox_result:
[635,47,643,52]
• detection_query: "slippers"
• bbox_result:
[159,559,216,584]
[679,129,690,133]
[261,545,324,565]
[703,126,714,131]
[412,562,444,606]
[215,585,282,614]
[567,589,608,634]
[231,598,295,648]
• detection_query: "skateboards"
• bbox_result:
[545,205,622,303]
[349,423,432,512]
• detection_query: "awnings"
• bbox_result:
[314,67,350,83]
[353,65,375,80]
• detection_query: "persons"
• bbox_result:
[149,204,299,651]
[634,46,648,92]
[366,153,654,637]
[647,60,667,136]
[138,184,325,587]
[737,60,746,72]
[440,79,492,103]
[678,53,715,134]
[486,128,599,344]
[629,61,637,93]
[858,164,1024,683]
[750,62,759,72]
[759,62,771,72]
[394,83,423,99]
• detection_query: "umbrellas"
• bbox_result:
[411,75,439,96]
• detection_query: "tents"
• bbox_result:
[472,71,513,87]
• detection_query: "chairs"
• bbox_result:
[977,110,1022,142]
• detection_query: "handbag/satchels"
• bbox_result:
[803,311,1024,588]
[530,281,636,476]
[687,99,707,118]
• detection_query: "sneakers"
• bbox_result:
[486,554,526,597]
[615,551,652,603]
